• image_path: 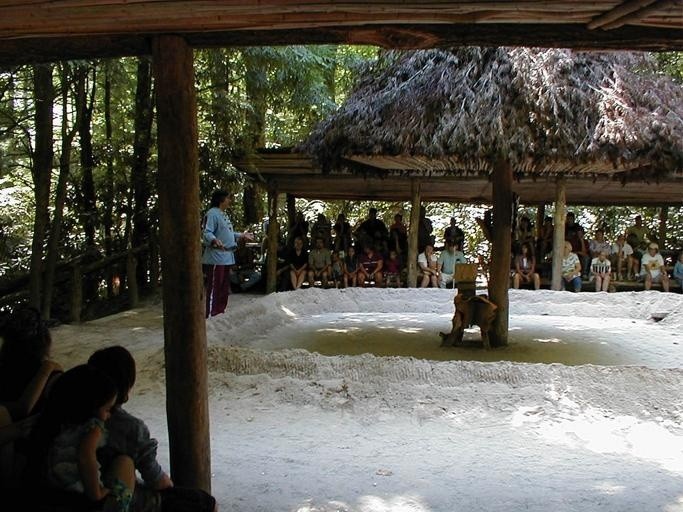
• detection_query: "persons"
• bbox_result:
[230,205,683,293]
[82,273,99,306]
[201,190,253,318]
[1,310,217,511]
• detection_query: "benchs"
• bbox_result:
[510,249,682,288]
[302,245,445,287]
